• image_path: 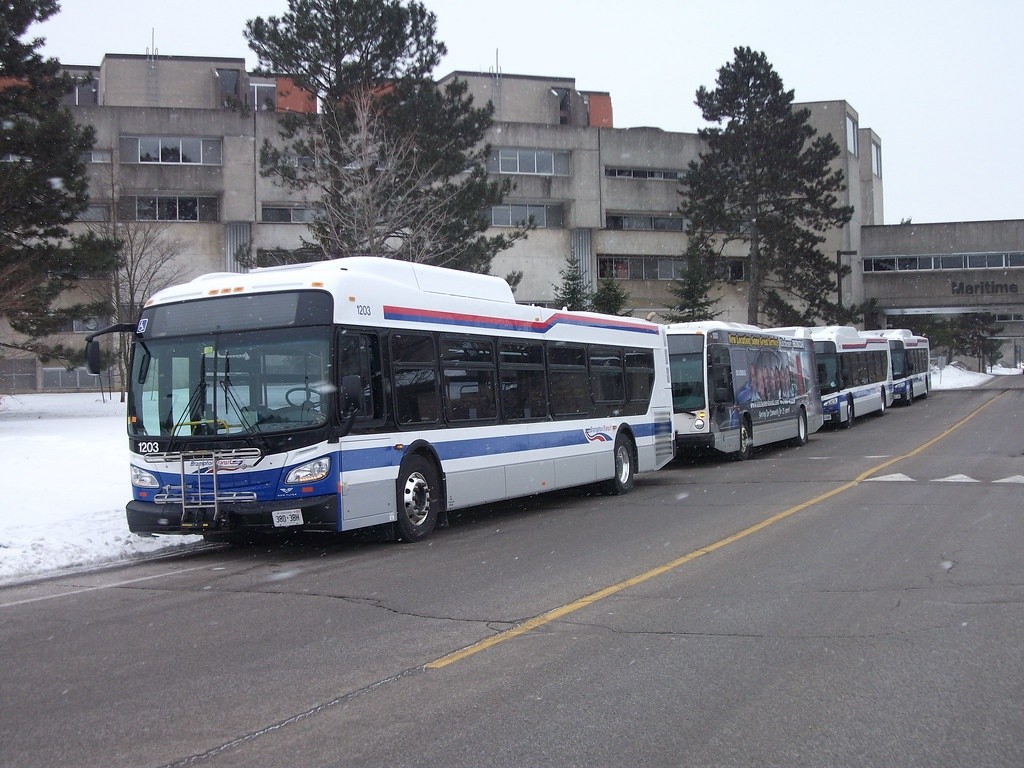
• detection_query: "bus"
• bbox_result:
[862,328,932,406]
[810,325,895,429]
[82,258,680,544]
[663,320,823,460]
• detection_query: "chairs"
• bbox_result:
[417,394,455,421]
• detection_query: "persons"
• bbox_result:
[748,363,798,402]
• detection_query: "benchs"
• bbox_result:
[459,384,506,401]
